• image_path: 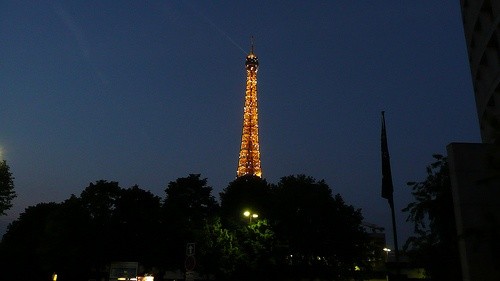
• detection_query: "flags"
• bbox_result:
[380,113,394,208]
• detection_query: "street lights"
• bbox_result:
[243,209,260,226]
[382,247,392,281]
